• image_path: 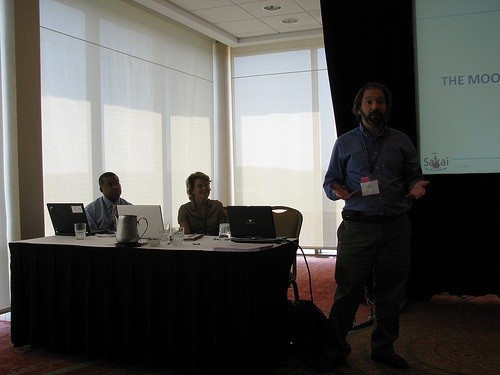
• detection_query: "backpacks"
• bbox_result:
[295,299,352,357]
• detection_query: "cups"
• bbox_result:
[158,224,170,247]
[74,223,86,240]
[218,223,232,239]
[171,227,184,248]
[148,237,160,246]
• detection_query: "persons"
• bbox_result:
[323,83,429,368]
[178,172,225,235]
[85,173,130,234]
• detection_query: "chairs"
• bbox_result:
[271,206,304,301]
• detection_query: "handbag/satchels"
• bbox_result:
[286,299,307,355]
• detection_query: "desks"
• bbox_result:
[8,235,299,375]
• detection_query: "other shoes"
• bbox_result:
[314,355,346,373]
[371,342,408,369]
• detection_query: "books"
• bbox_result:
[214,243,274,252]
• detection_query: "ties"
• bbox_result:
[111,203,117,231]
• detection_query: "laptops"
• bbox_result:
[117,204,170,240]
[47,203,107,236]
[227,206,287,242]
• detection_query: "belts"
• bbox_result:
[358,213,405,225]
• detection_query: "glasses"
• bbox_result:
[194,184,209,189]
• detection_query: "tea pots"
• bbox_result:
[113,214,149,244]
